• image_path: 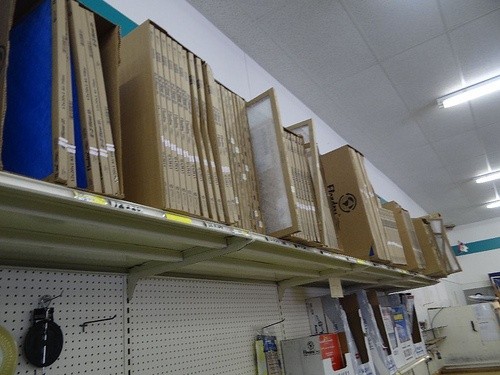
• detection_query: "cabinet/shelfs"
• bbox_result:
[421,325,446,364]
[0,172,440,296]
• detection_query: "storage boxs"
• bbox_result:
[267,290,427,375]
[0,0,465,279]
